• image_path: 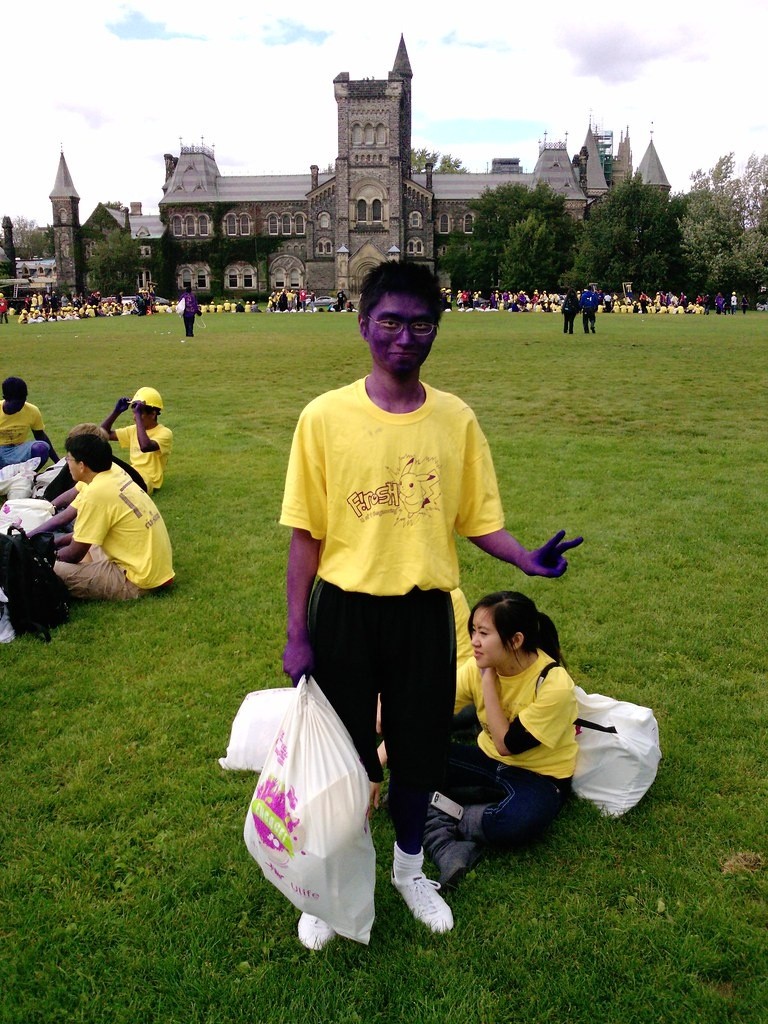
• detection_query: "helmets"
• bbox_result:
[126,385,163,410]
[0,293,4,298]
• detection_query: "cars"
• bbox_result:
[478,297,491,308]
[154,296,172,307]
[314,295,337,307]
[305,294,312,306]
[101,295,136,303]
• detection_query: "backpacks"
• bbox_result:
[562,295,578,314]
[0,524,73,641]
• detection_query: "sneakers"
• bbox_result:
[390,868,454,934]
[298,912,336,950]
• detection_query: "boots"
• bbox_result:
[455,802,498,845]
[421,792,489,893]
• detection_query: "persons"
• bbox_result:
[177,286,198,337]
[264,287,364,314]
[16,422,176,604]
[0,288,263,325]
[276,258,588,952]
[101,386,175,498]
[1,375,59,488]
[438,282,768,336]
[362,588,581,898]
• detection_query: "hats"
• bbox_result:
[19,282,360,326]
[442,287,735,307]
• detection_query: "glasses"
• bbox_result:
[365,314,438,336]
[65,454,88,468]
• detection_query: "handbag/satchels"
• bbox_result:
[0,499,56,536]
[243,670,377,946]
[176,293,186,318]
[0,457,41,499]
[536,661,662,820]
[31,457,67,499]
